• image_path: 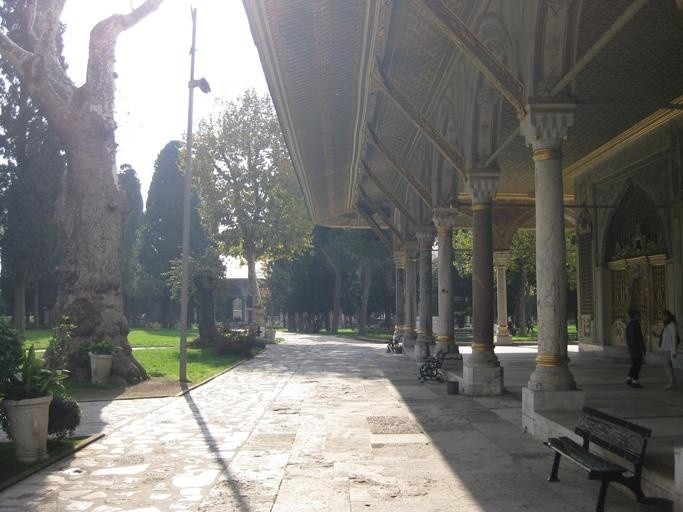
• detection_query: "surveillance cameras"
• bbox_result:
[189,77,211,94]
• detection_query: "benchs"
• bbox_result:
[542,405,652,510]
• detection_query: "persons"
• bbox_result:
[526,318,533,333]
[657,310,679,388]
[625,309,645,388]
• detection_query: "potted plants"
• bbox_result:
[0,345,70,463]
[78,336,123,385]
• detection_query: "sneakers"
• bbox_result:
[626,379,643,389]
[664,384,680,392]
